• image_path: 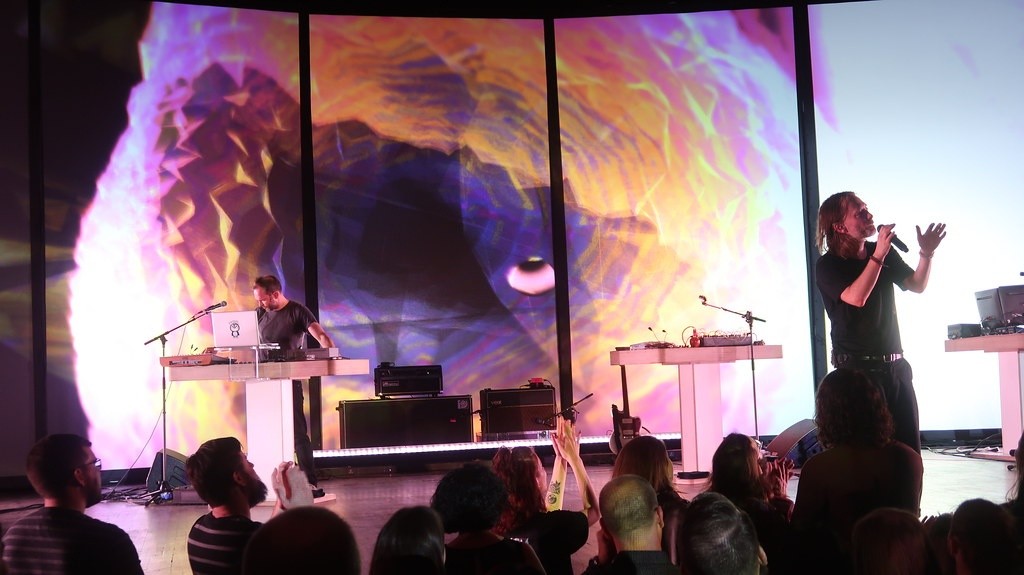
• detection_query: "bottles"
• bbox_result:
[690,329,700,347]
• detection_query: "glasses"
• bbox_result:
[70,458,101,475]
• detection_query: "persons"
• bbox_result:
[185,437,315,575]
[706,433,795,575]
[368,505,446,575]
[202,275,335,490]
[584,474,676,575]
[243,505,360,575]
[851,431,1024,575]
[0,433,144,575]
[815,191,946,454]
[429,463,547,575]
[790,368,924,575]
[491,415,600,575]
[612,436,690,565]
[674,492,768,575]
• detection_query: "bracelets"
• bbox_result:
[919,251,934,258]
[870,256,883,266]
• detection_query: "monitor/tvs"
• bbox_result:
[975,285,1024,326]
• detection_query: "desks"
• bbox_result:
[944,333,1024,461]
[169,358,369,506]
[612,345,782,483]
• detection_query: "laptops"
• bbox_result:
[212,311,279,347]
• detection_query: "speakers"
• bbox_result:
[480,387,557,434]
[338,394,474,450]
[145,448,189,496]
[765,419,827,468]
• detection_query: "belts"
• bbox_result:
[836,353,903,364]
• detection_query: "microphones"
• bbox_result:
[877,224,909,252]
[536,418,556,427]
[198,301,227,314]
